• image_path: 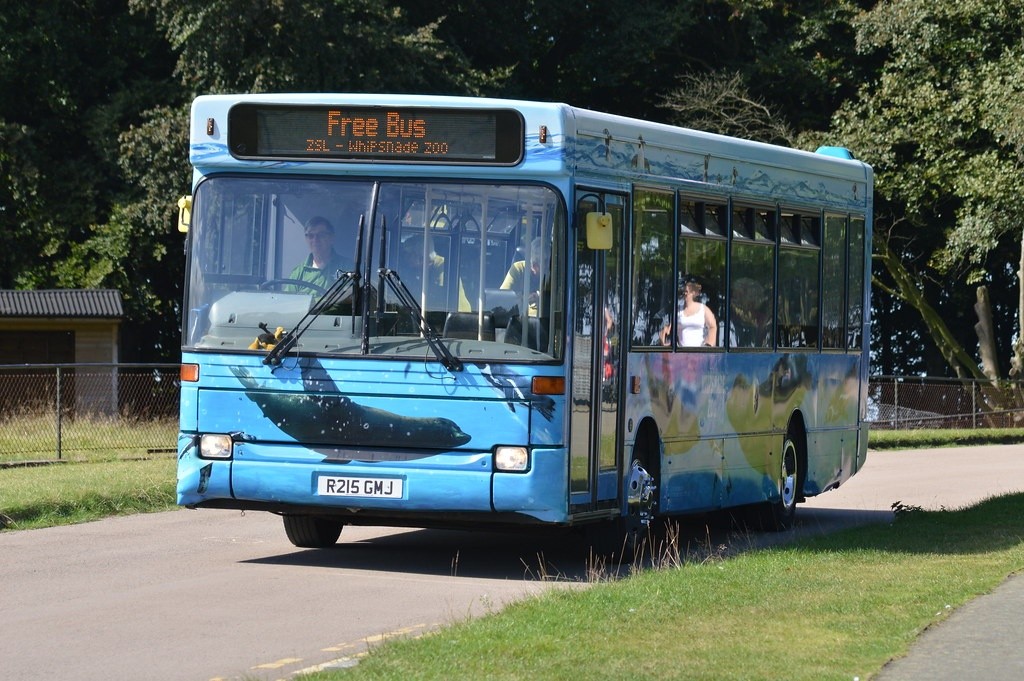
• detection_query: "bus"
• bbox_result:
[172,88,879,561]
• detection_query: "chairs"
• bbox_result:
[442,310,498,343]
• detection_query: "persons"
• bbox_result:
[402,233,471,313]
[660,283,717,347]
[498,236,553,353]
[282,216,360,317]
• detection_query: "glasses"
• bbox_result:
[304,232,333,239]
